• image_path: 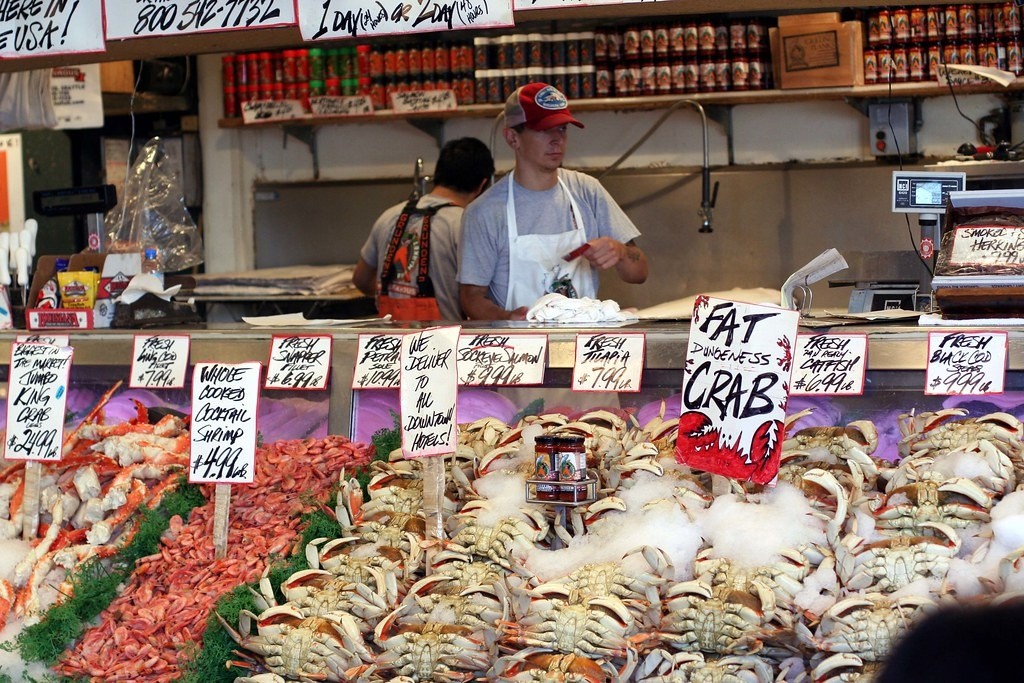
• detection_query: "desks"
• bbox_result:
[174,287,369,321]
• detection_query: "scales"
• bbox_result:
[847,170,966,316]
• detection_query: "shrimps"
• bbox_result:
[55,434,375,683]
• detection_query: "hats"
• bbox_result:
[504,82,584,131]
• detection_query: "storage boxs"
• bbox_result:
[769,11,863,88]
[26,251,142,331]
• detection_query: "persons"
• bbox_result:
[351,137,496,320]
[456,82,648,321]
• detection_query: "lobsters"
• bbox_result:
[0,380,200,629]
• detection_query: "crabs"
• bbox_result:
[214,402,1023,683]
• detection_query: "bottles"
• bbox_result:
[142,249,164,286]
